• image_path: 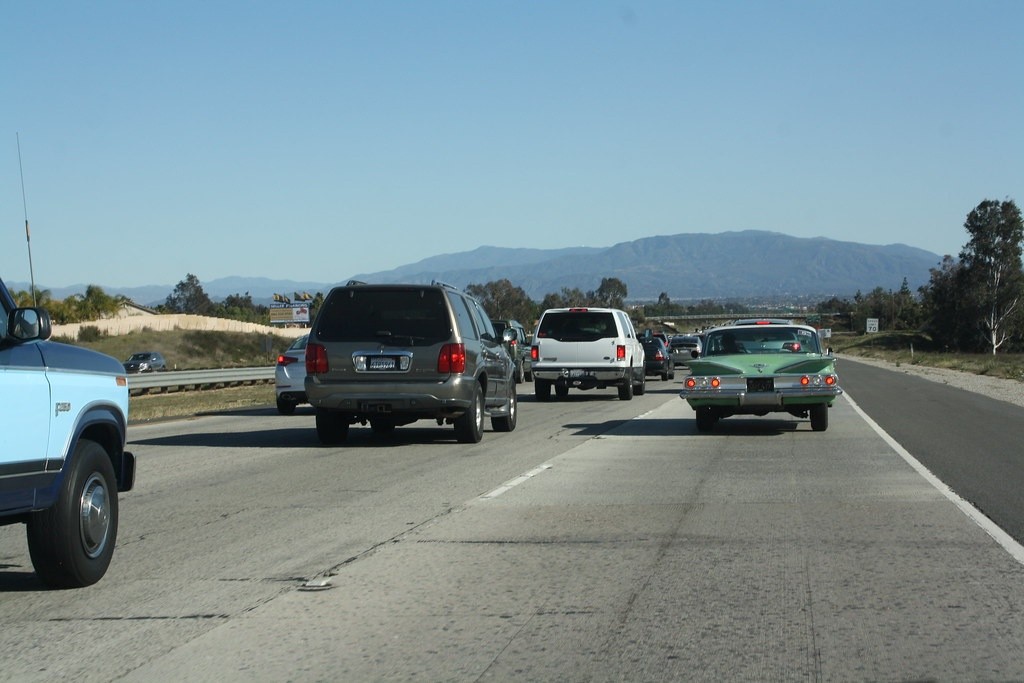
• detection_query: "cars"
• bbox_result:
[638,337,675,381]
[123,352,167,374]
[666,336,702,365]
[679,325,843,432]
[274,334,309,415]
[722,319,805,353]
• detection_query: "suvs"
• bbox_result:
[0,278,137,591]
[305,279,518,443]
[531,307,645,400]
[491,318,534,384]
